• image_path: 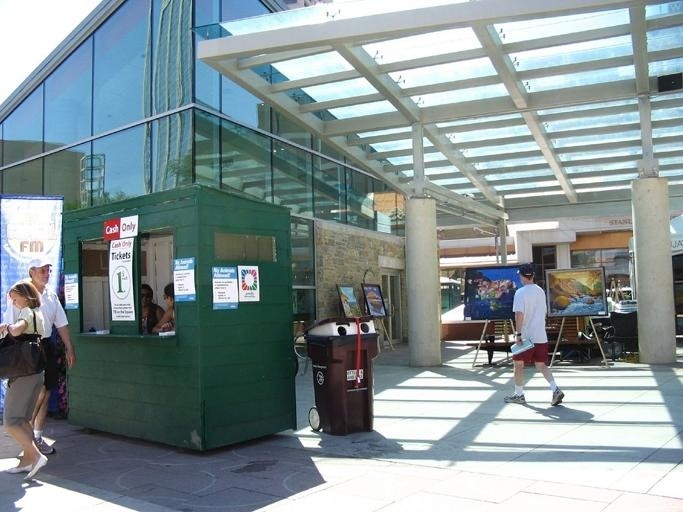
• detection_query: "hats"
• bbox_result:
[517,263,534,278]
[27,258,53,270]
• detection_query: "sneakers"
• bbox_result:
[32,438,55,455]
[551,388,565,406]
[503,393,525,404]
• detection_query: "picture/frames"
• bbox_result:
[336,284,363,319]
[462,262,536,321]
[543,266,608,318]
[360,282,388,320]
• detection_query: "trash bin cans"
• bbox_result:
[303,313,380,436]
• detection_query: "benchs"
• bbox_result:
[463,316,605,365]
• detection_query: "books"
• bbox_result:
[510,338,534,356]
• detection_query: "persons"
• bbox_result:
[504,263,564,406]
[6,258,76,454]
[0,281,48,480]
[154,283,174,333]
[140,284,165,333]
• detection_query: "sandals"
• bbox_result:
[24,455,48,480]
[6,463,32,474]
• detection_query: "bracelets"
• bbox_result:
[515,333,522,336]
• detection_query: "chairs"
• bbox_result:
[604,310,639,361]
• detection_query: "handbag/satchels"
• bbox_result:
[0,332,48,379]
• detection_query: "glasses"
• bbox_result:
[162,294,170,300]
